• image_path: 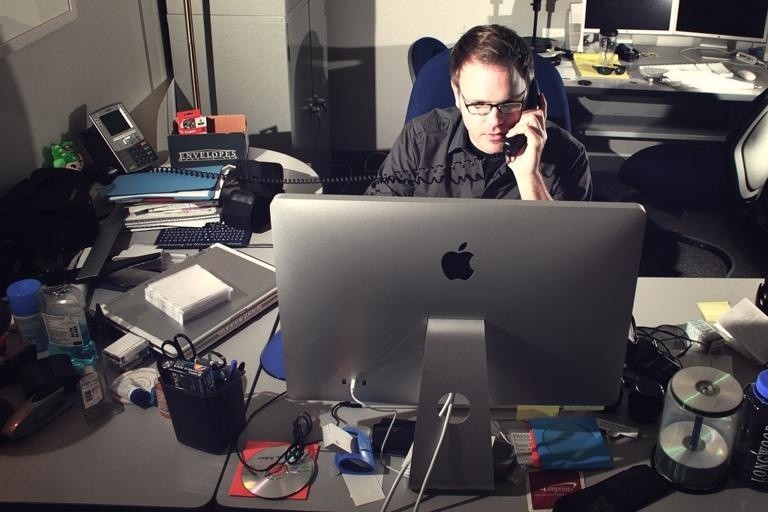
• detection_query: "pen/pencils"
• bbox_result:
[193,360,245,391]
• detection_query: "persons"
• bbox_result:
[366,25,595,202]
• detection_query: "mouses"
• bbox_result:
[736,70,757,82]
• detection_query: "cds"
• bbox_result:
[242,445,314,500]
[111,368,160,404]
[653,365,744,494]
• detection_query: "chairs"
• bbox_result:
[407,36,448,85]
[617,87,768,278]
[404,46,571,139]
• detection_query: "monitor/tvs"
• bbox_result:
[579,0,675,55]
[673,0,768,65]
[269,193,647,495]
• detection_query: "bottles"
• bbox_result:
[5,278,49,345]
[729,368,768,493]
[595,25,619,66]
[75,365,114,419]
[34,266,99,371]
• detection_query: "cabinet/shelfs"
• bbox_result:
[209,0,333,178]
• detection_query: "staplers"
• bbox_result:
[2,383,76,440]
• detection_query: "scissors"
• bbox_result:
[199,350,227,369]
[162,334,196,362]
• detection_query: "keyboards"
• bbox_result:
[153,224,253,249]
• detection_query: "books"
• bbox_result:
[144,263,234,324]
[712,297,768,367]
[106,161,238,233]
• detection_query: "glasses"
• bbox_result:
[451,78,527,118]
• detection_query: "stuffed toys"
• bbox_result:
[50,140,85,172]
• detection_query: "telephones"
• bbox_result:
[503,78,541,156]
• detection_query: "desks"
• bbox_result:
[543,45,767,143]
[1,145,325,510]
[214,278,768,510]
